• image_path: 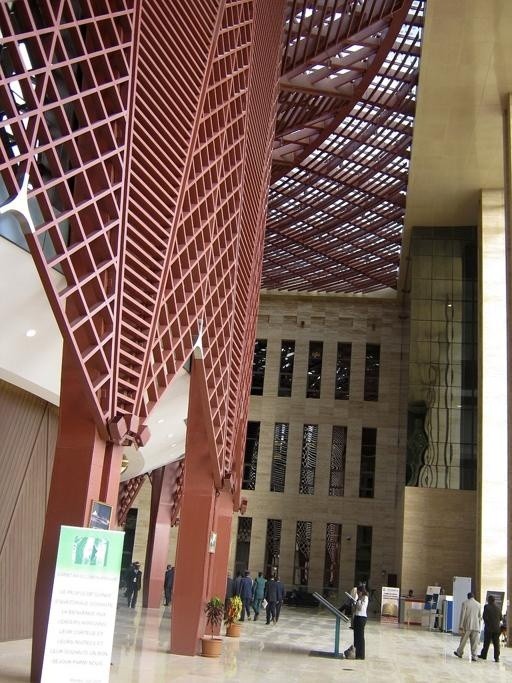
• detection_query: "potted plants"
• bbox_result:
[198,596,225,661]
[223,594,244,642]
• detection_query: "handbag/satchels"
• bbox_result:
[344,645,356,659]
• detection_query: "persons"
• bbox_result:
[452,593,482,661]
[350,580,369,660]
[407,588,414,599]
[224,571,285,625]
[477,596,503,661]
[123,561,176,609]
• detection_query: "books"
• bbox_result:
[344,592,357,605]
